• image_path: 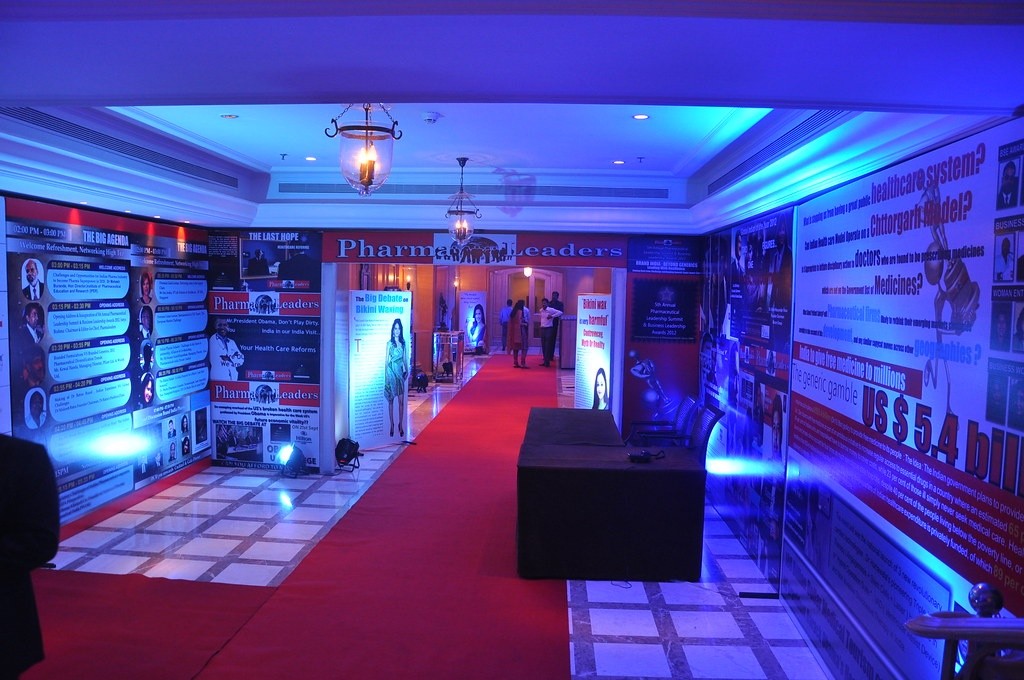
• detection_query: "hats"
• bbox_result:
[144,342,153,354]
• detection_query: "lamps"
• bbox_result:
[417,372,428,393]
[279,442,308,479]
[325,103,402,195]
[475,346,483,355]
[442,362,453,376]
[335,438,361,472]
[445,157,482,245]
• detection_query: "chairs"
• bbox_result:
[625,394,725,469]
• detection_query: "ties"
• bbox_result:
[257,257,260,260]
[147,333,150,339]
[33,288,39,301]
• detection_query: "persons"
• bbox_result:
[136,271,156,404]
[22,302,43,343]
[699,215,793,590]
[207,318,245,380]
[466,303,487,348]
[0,434,61,680]
[248,241,319,291]
[384,319,408,436]
[136,415,192,478]
[500,291,565,369]
[215,424,266,462]
[590,369,611,410]
[16,387,47,429]
[22,260,44,300]
[19,350,45,387]
[986,163,1024,432]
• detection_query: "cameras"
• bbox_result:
[628,450,651,463]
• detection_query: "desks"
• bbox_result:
[517,407,708,582]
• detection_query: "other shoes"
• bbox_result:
[398,422,404,437]
[390,422,394,437]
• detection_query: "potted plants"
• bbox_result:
[440,293,448,327]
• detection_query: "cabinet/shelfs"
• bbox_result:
[434,331,465,382]
